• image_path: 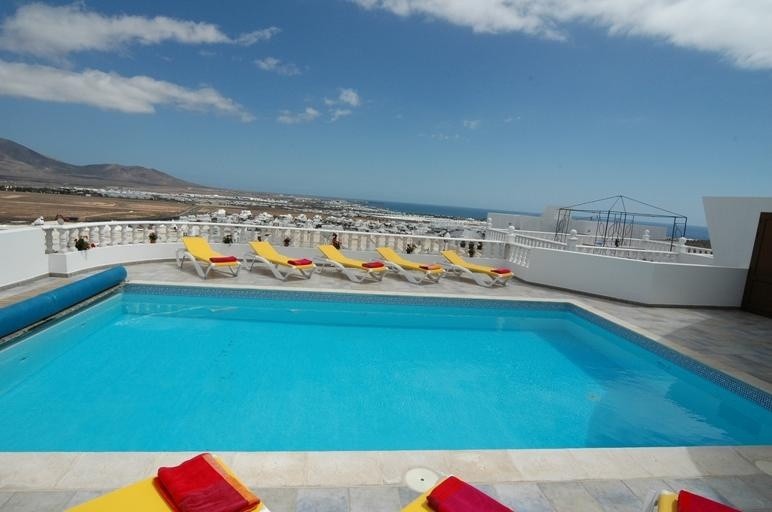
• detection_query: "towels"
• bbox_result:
[676,490,742,512]
[428,476,516,512]
[154,453,262,512]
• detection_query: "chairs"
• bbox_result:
[242,240,317,281]
[370,246,446,285]
[313,243,389,283]
[176,235,243,280]
[436,250,515,288]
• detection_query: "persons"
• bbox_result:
[460,241,482,257]
[615,235,620,248]
